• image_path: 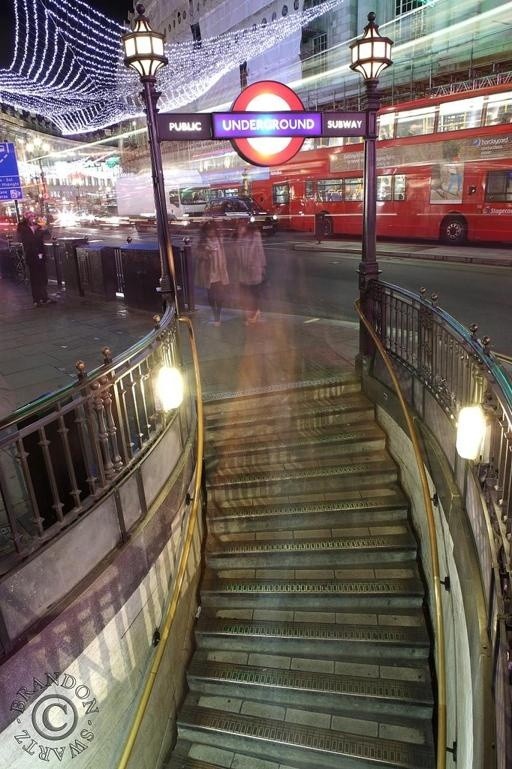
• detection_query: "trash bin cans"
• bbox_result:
[18,394,90,529]
[53,238,183,312]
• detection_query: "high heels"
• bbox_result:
[240,309,261,326]
[207,312,222,327]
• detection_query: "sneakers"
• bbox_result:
[33,298,58,306]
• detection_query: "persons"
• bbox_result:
[17,210,57,306]
[230,217,265,327]
[195,217,234,332]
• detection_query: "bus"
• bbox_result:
[0,81,511,241]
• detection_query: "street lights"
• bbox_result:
[25,135,53,239]
[348,11,396,377]
[118,4,188,394]
